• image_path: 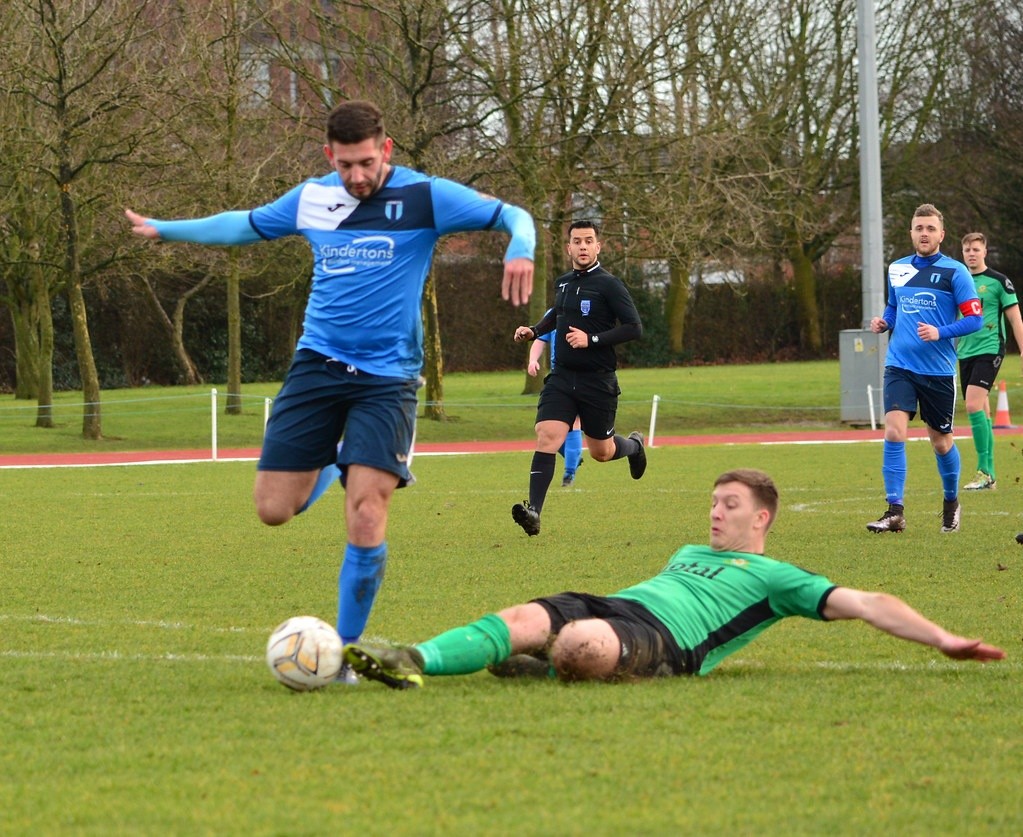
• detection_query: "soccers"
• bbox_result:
[266,616,343,692]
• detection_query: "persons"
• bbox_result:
[861,203,986,533]
[953,231,1023,491]
[348,468,1009,689]
[522,305,586,487]
[511,221,648,537]
[115,102,538,689]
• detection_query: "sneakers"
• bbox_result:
[980,479,997,488]
[559,473,574,490]
[330,643,361,685]
[482,655,556,680]
[964,472,990,488]
[510,500,541,535]
[627,430,648,480]
[341,635,424,688]
[575,457,583,467]
[867,509,907,532]
[939,502,962,533]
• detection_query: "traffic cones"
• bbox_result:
[990,378,1018,429]
